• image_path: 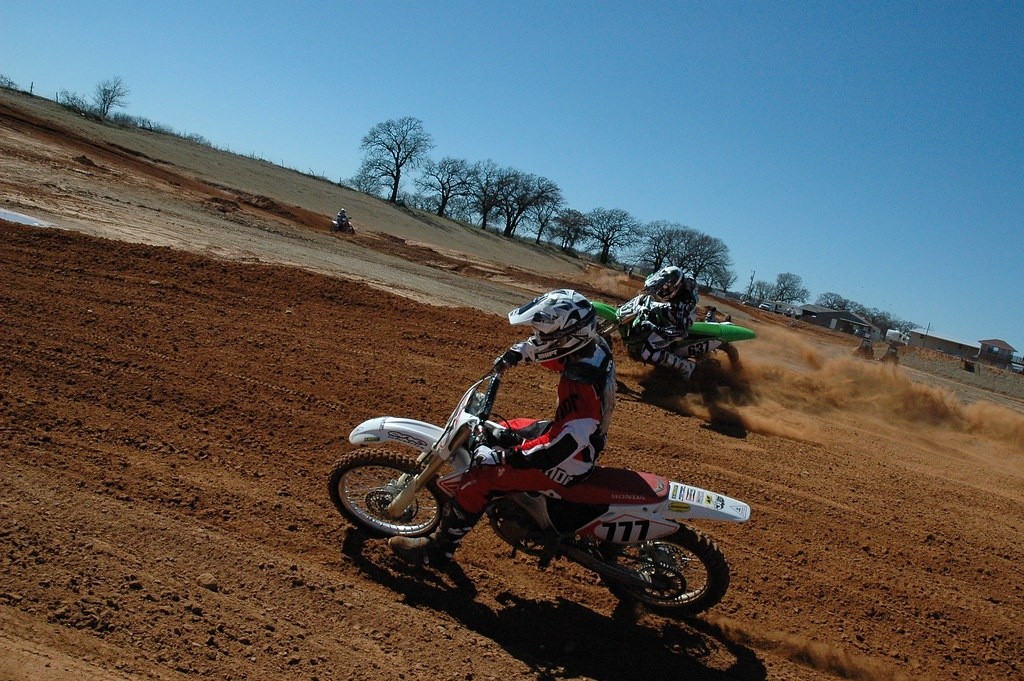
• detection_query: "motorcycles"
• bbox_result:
[328,349,751,616]
[330,217,355,235]
[589,301,755,379]
[852,338,906,367]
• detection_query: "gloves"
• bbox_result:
[503,349,523,368]
[641,320,657,332]
[472,445,501,470]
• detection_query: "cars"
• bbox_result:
[759,302,772,311]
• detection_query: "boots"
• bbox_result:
[655,350,696,382]
[389,498,481,573]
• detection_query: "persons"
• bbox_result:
[389,288,617,573]
[337,209,355,232]
[634,265,703,384]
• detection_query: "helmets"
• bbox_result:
[642,265,683,301]
[507,288,596,364]
